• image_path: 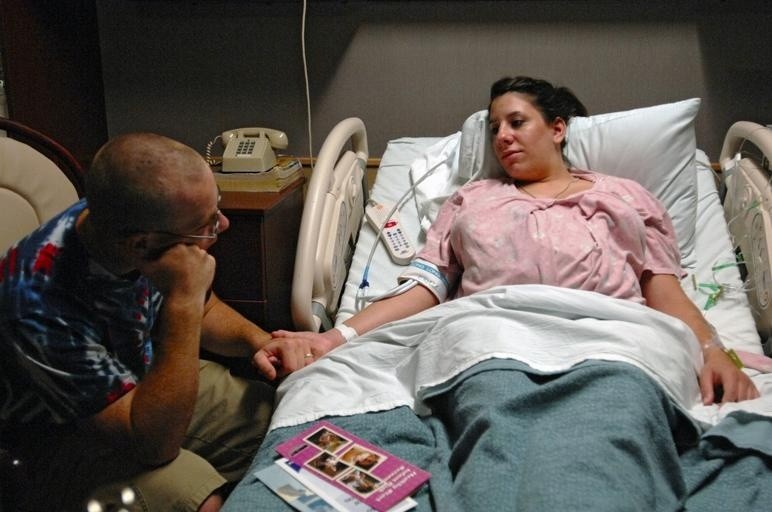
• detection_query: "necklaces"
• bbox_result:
[521,177,578,198]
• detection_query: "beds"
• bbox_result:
[218,117,772,512]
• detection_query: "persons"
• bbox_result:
[267,75,761,512]
[2,131,315,511]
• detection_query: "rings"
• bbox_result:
[304,353,313,358]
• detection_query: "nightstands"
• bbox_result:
[213,175,307,327]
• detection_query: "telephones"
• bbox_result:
[222,127,288,173]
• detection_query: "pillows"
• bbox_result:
[458,96,702,268]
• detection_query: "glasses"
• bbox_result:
[120,184,223,240]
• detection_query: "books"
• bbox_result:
[210,156,302,194]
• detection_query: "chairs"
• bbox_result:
[0,115,87,263]
